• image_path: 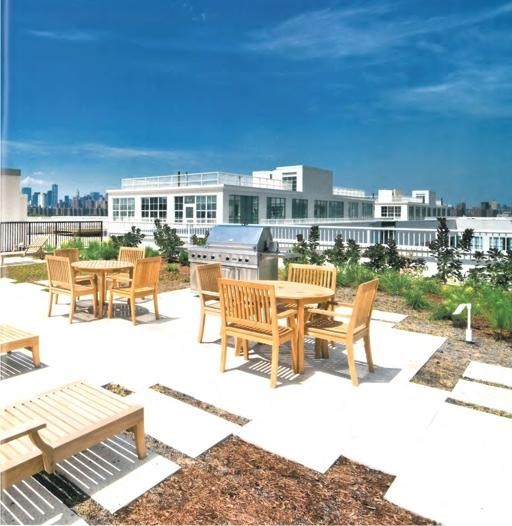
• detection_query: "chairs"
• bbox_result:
[195,262,379,388]
[0,379,146,490]
[0,324,40,368]
[45,247,162,326]
